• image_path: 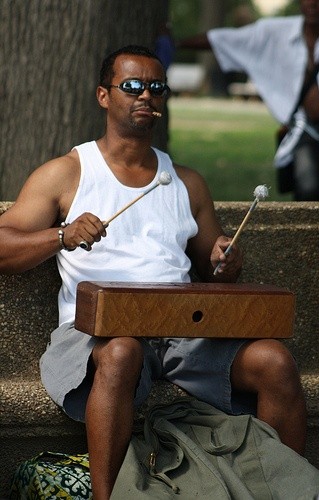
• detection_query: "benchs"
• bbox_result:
[0,200,318,438]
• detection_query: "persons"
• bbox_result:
[0,45,308,500]
[179,0,319,201]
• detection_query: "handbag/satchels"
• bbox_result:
[111,397,319,500]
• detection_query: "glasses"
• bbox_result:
[106,77,169,98]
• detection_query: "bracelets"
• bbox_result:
[58,222,77,252]
